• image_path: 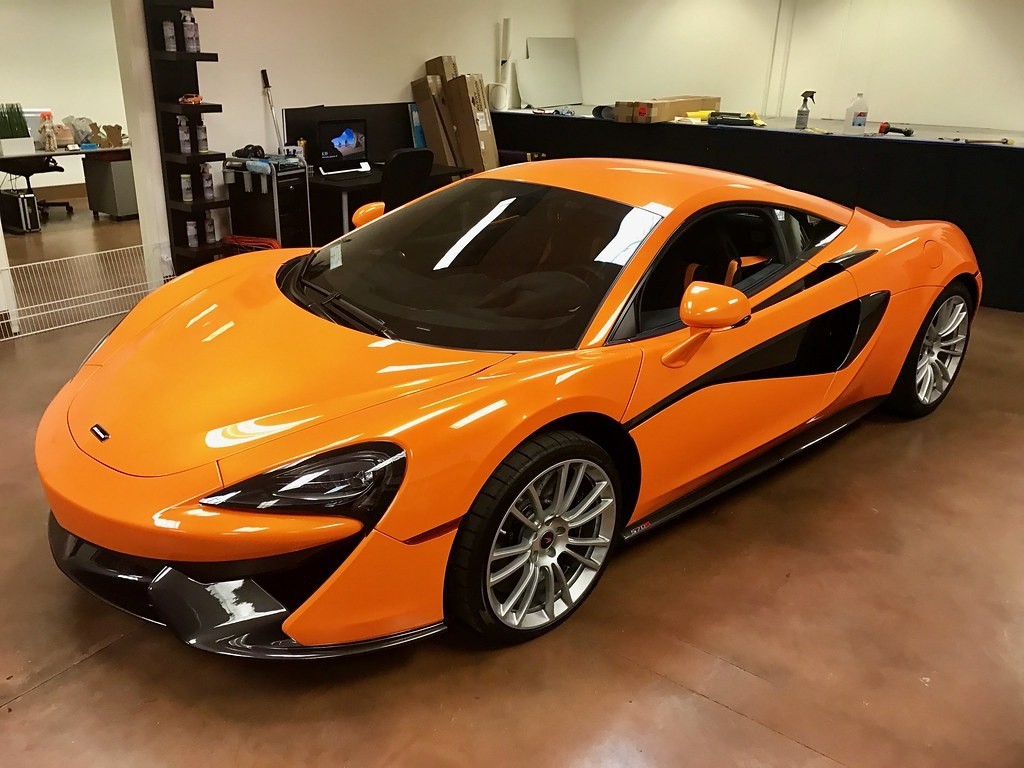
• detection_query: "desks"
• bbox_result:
[1,142,133,219]
[307,155,475,249]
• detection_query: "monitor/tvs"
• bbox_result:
[316,119,368,163]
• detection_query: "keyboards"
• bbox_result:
[323,170,371,182]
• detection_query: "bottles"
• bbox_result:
[844,93,869,135]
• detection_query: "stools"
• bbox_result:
[2,158,74,219]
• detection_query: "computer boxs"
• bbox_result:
[223,152,313,247]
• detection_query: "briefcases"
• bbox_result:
[1,187,40,234]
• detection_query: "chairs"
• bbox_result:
[382,148,435,211]
[670,226,741,302]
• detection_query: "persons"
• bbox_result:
[38,111,58,153]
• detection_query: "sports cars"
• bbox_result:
[32,155,982,664]
